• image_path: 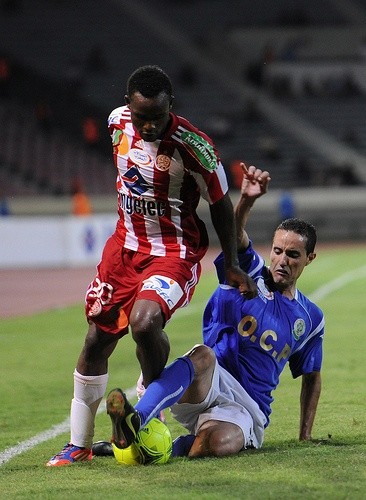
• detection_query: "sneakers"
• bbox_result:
[45,444,93,466]
[92,440,114,456]
[106,388,140,449]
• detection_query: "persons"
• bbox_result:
[45,65,259,467]
[90,160,327,463]
[0,181,310,246]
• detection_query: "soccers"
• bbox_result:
[111,417,173,464]
